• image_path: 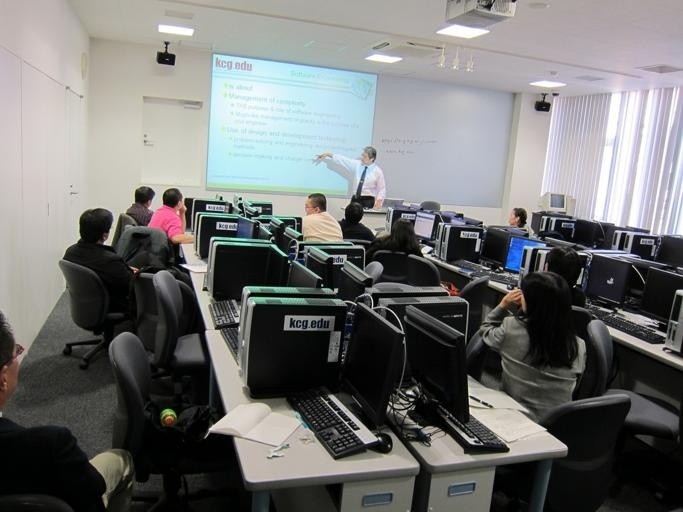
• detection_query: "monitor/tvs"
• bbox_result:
[237,215,260,238]
[586,255,632,309]
[463,217,483,226]
[286,260,323,288]
[545,236,590,251]
[267,244,289,286]
[340,301,406,430]
[233,194,242,207]
[216,193,223,201]
[245,207,258,219]
[242,200,253,215]
[638,265,683,324]
[224,202,233,214]
[337,260,374,312]
[538,192,567,214]
[480,228,512,266]
[619,255,673,291]
[503,235,549,274]
[413,211,437,243]
[306,247,334,292]
[401,305,470,424]
[270,217,286,251]
[283,227,305,262]
[507,228,529,237]
[654,235,683,268]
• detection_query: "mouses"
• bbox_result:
[507,284,514,290]
[593,244,598,249]
[367,431,393,454]
[495,267,504,274]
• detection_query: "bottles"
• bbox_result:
[159,409,178,429]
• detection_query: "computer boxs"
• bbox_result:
[440,224,483,262]
[547,217,577,238]
[238,200,273,215]
[183,197,194,230]
[584,249,627,254]
[434,221,454,257]
[206,236,272,291]
[259,214,303,234]
[303,244,366,288]
[623,233,659,259]
[191,198,227,233]
[364,286,451,311]
[251,217,297,232]
[663,289,683,355]
[195,211,238,253]
[292,240,354,266]
[611,229,634,250]
[196,214,238,259]
[208,241,270,301]
[517,245,555,289]
[539,216,552,232]
[385,207,424,234]
[600,253,642,258]
[238,285,338,377]
[377,295,470,346]
[534,250,589,289]
[242,296,348,399]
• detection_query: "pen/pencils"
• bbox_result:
[470,395,494,408]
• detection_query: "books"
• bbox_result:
[209,402,302,447]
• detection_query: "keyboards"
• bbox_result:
[438,405,510,453]
[220,327,239,366]
[597,314,666,344]
[451,258,491,273]
[208,299,240,330]
[470,270,518,287]
[586,303,611,318]
[286,385,379,460]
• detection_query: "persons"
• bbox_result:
[479,271,586,424]
[315,147,386,209]
[147,188,195,244]
[545,245,586,308]
[338,202,375,241]
[509,207,527,229]
[63,208,139,317]
[0,314,134,512]
[365,218,424,266]
[126,187,154,227]
[302,193,343,242]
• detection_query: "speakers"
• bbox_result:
[534,100,551,112]
[156,51,176,66]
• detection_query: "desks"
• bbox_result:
[179,228,208,264]
[388,374,568,511]
[207,328,420,512]
[423,253,472,279]
[187,269,242,329]
[467,272,518,294]
[603,324,683,371]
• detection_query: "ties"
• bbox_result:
[355,166,368,199]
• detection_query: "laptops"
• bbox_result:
[373,198,405,210]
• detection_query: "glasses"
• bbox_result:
[1,343,24,367]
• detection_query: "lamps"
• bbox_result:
[435,55,446,69]
[364,51,404,64]
[465,60,475,73]
[529,79,568,88]
[157,22,196,37]
[449,57,460,71]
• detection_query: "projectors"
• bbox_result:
[443,1,518,29]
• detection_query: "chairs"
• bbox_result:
[133,269,197,352]
[152,270,207,370]
[108,332,222,511]
[343,239,371,250]
[58,259,151,370]
[572,320,681,439]
[373,250,441,288]
[537,393,631,464]
[457,275,490,307]
